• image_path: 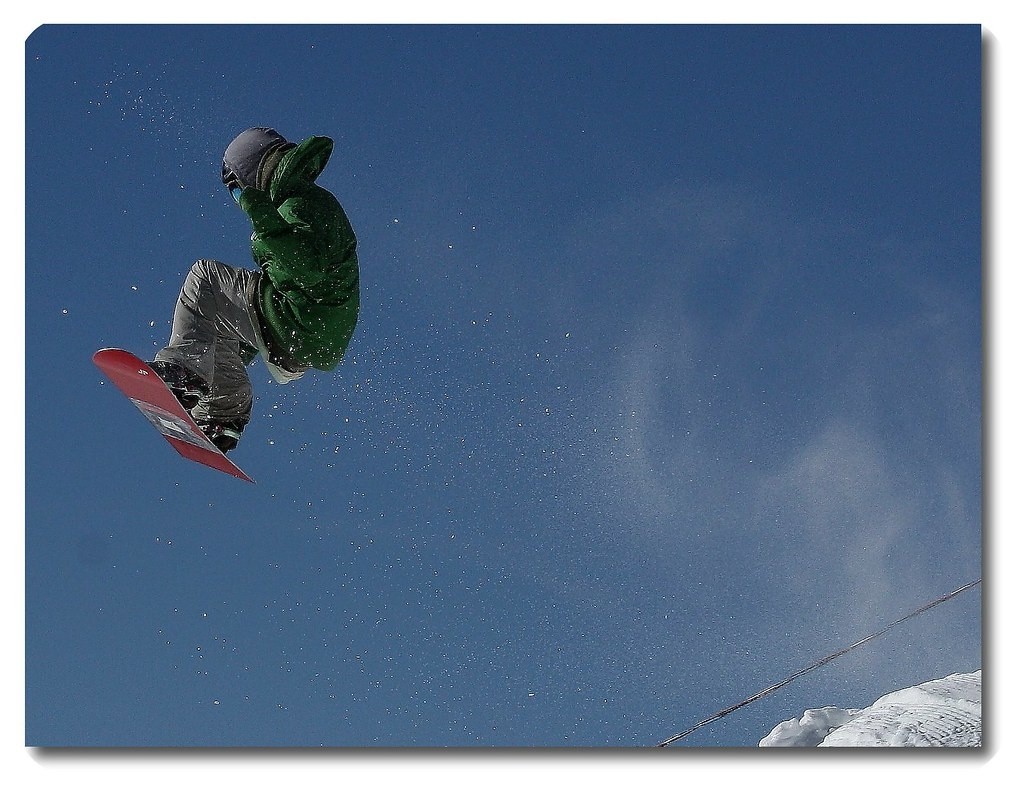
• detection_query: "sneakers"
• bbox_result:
[195,420,243,452]
[146,361,209,407]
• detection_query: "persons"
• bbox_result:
[145,127,361,450]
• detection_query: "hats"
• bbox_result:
[221,159,235,183]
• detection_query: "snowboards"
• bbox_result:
[92,343,251,484]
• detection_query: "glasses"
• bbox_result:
[228,180,242,204]
